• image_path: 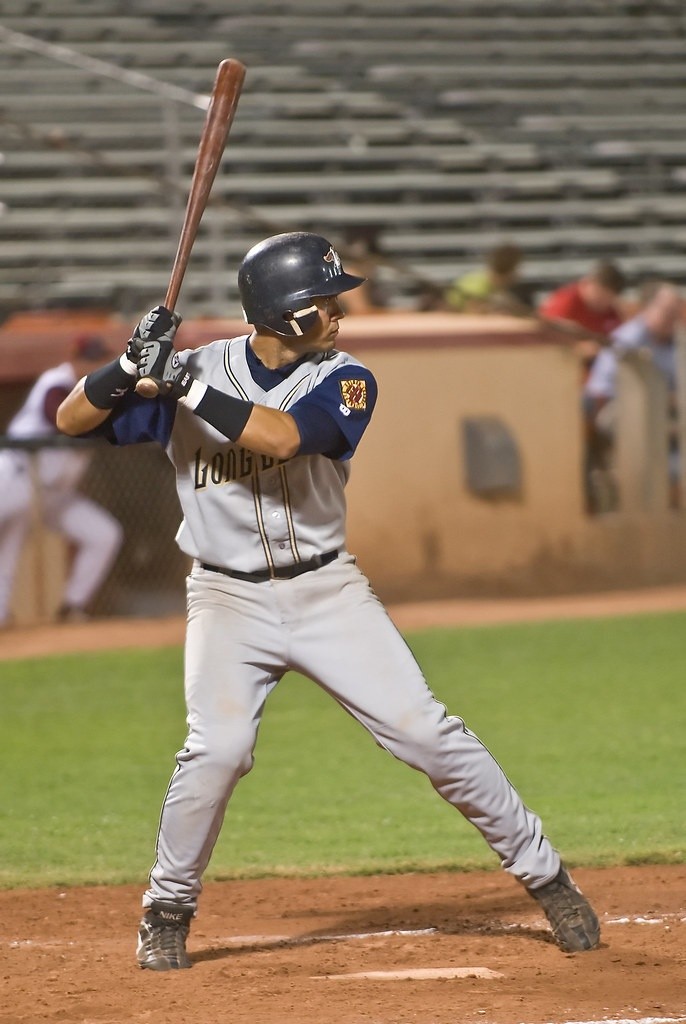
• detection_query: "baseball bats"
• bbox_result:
[134,56,247,397]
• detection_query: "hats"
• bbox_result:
[72,334,117,362]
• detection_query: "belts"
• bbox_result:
[200,549,338,583]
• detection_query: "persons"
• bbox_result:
[55,233,599,970]
[0,335,123,632]
[448,246,686,510]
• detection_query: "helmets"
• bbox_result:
[238,232,368,336]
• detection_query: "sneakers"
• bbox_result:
[525,858,601,953]
[136,900,197,971]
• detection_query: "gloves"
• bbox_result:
[125,305,182,364]
[137,340,194,400]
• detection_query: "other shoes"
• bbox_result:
[56,606,93,625]
